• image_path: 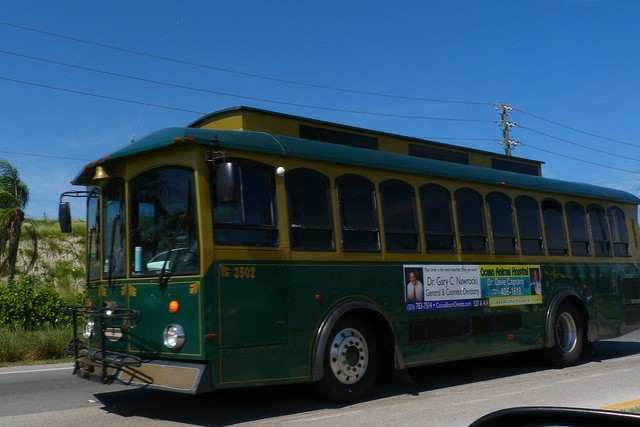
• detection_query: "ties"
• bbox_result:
[413,286,417,301]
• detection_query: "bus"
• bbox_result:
[58,105,639,402]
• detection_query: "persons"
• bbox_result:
[407,271,423,304]
[530,269,541,295]
